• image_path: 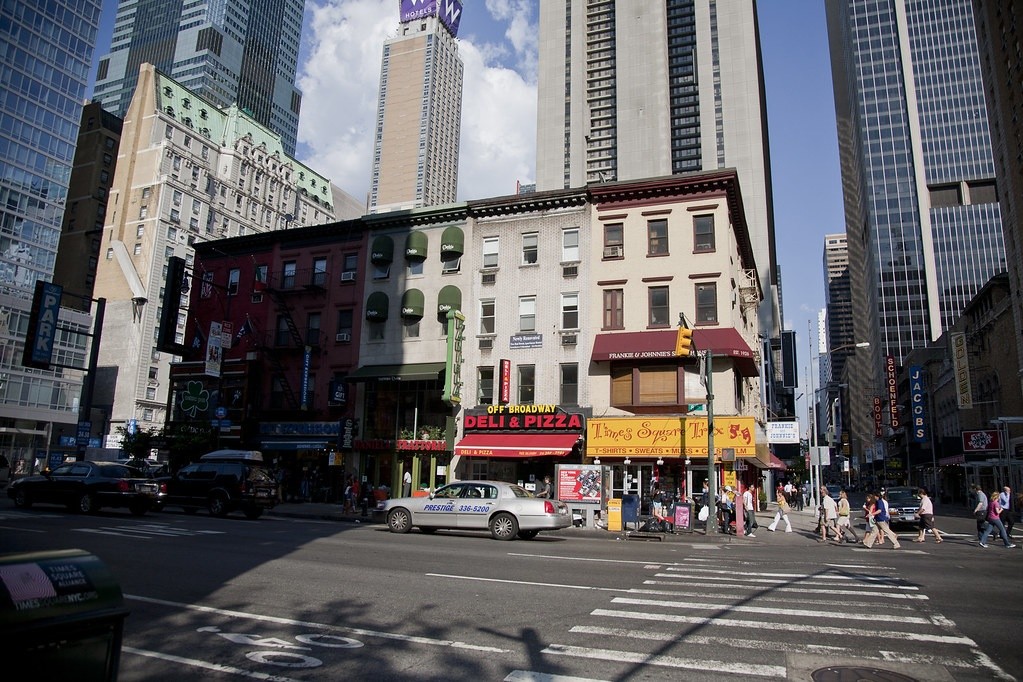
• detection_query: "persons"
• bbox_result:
[777,481,807,511]
[702,479,734,534]
[817,486,901,549]
[344,474,359,514]
[768,490,792,532]
[403,472,411,498]
[34,458,40,473]
[538,476,554,499]
[743,484,756,537]
[913,489,943,543]
[652,482,662,515]
[973,486,1017,548]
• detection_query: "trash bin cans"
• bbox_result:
[1,549,130,682]
[623,495,640,530]
[608,498,622,531]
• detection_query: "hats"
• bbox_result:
[724,486,732,491]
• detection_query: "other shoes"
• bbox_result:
[912,539,924,543]
[747,533,756,538]
[1006,544,1016,549]
[979,542,988,548]
[935,538,942,544]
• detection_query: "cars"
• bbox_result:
[371,480,571,541]
[111,459,164,478]
[7,460,168,516]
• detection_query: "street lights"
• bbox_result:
[814,383,849,504]
[808,319,870,523]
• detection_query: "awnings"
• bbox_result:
[770,453,786,470]
[455,434,583,457]
[344,363,446,381]
[592,328,760,376]
[365,286,461,321]
[371,227,464,263]
[261,442,328,448]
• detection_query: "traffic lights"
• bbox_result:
[675,326,693,357]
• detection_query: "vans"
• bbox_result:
[0,454,11,490]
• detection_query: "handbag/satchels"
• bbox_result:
[975,510,988,520]
[780,503,791,514]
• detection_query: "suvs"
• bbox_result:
[881,487,935,533]
[823,484,844,506]
[167,449,280,521]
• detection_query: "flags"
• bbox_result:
[192,319,252,348]
